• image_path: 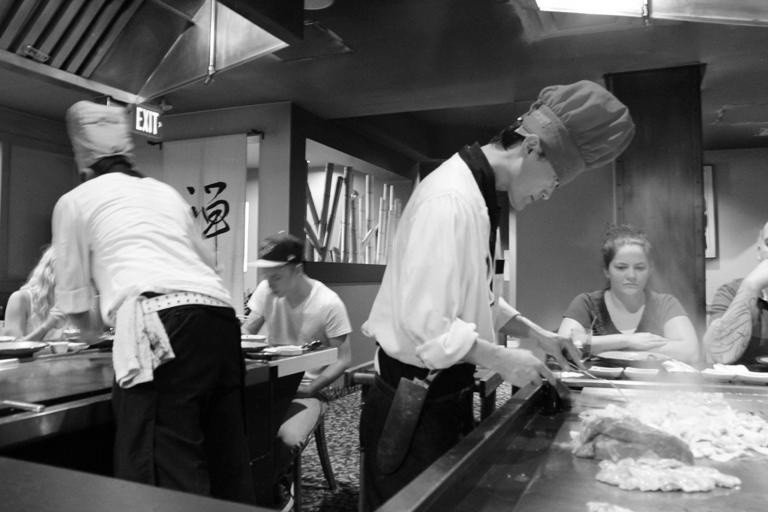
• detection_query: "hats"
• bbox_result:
[246,232,305,269]
[515,80,638,185]
[64,99,136,172]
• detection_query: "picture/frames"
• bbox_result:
[701,163,718,259]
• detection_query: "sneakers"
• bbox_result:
[274,472,296,512]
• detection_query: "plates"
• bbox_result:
[701,367,736,382]
[0,341,49,355]
[738,371,768,385]
[624,366,659,379]
[241,333,266,342]
[597,351,668,366]
[241,342,267,352]
[588,365,624,377]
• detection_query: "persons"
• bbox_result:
[360,80,636,512]
[238,231,353,511]
[555,222,699,364]
[702,223,768,365]
[2,245,80,346]
[53,109,246,501]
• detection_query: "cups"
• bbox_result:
[51,343,68,354]
[568,327,593,363]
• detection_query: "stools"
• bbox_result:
[291,411,336,512]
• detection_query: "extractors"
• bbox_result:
[0,1,306,115]
